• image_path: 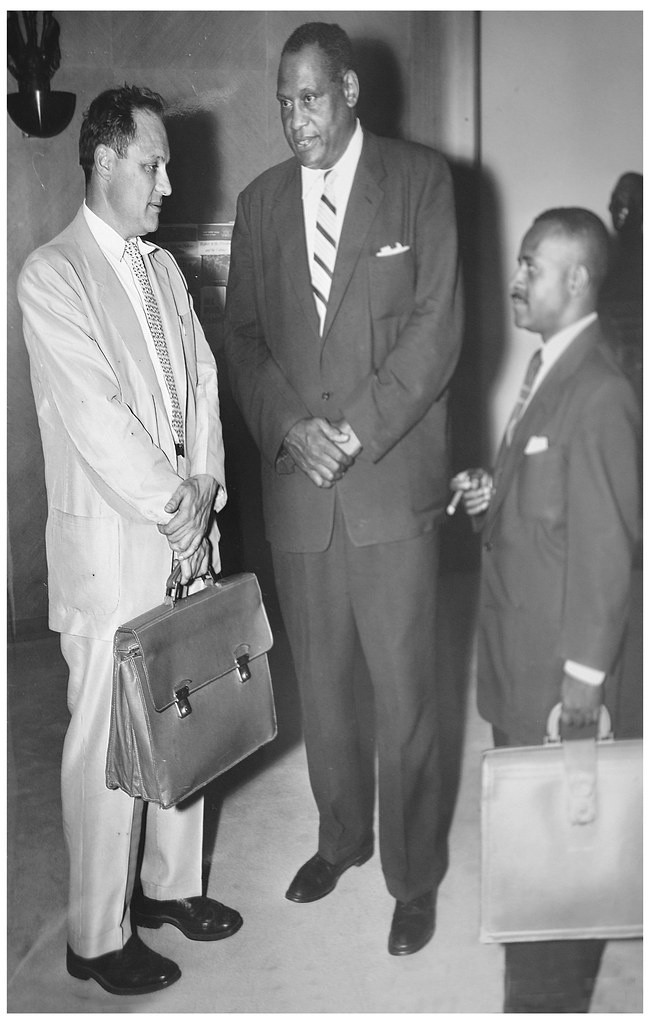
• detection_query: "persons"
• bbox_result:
[219,19,470,955]
[451,205,644,1014]
[14,84,247,998]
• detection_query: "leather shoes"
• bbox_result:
[388,889,437,957]
[285,839,375,902]
[65,935,184,996]
[131,886,243,942]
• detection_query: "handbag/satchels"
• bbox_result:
[476,699,643,946]
[103,559,280,810]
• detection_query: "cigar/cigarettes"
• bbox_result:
[444,490,464,515]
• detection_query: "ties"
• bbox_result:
[308,169,341,336]
[126,242,185,449]
[502,350,542,449]
